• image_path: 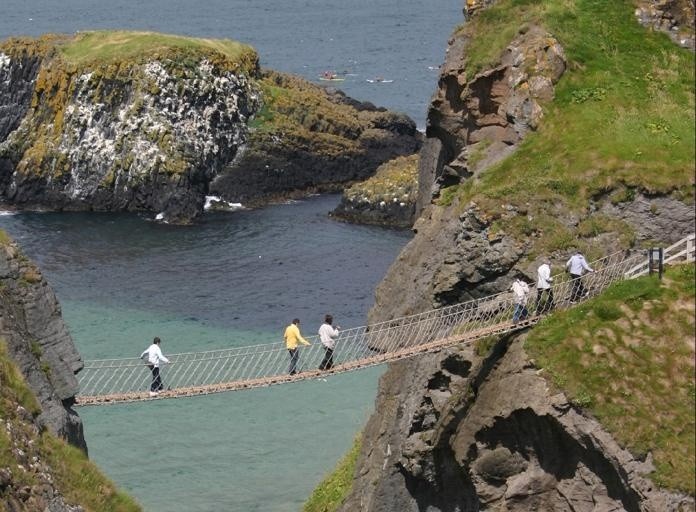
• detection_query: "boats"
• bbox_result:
[426,66,441,70]
[366,79,394,83]
[333,73,359,77]
[318,77,345,82]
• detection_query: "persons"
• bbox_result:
[563,249,595,304]
[534,258,557,317]
[138,335,172,397]
[316,314,342,371]
[507,273,531,324]
[283,317,312,377]
